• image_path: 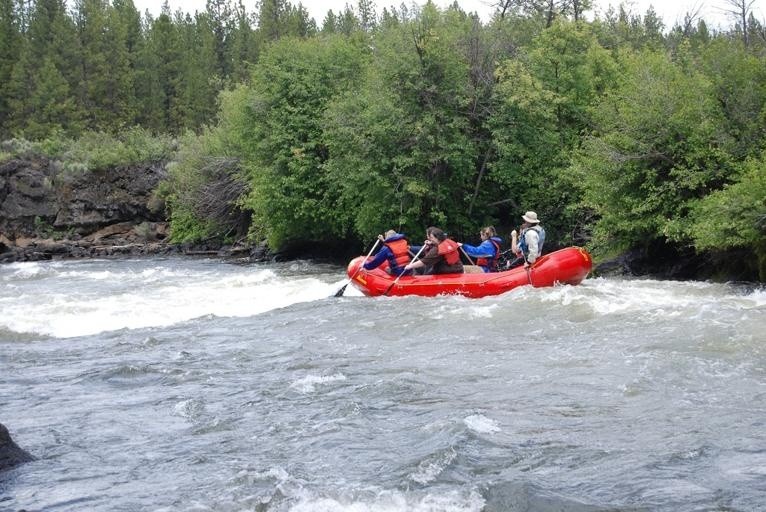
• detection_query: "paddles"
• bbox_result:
[333,234,380,298]
[382,241,428,295]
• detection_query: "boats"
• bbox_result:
[347,244,592,301]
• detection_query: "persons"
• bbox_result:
[359,210,546,275]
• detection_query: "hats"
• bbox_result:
[383,230,405,242]
[521,211,540,224]
